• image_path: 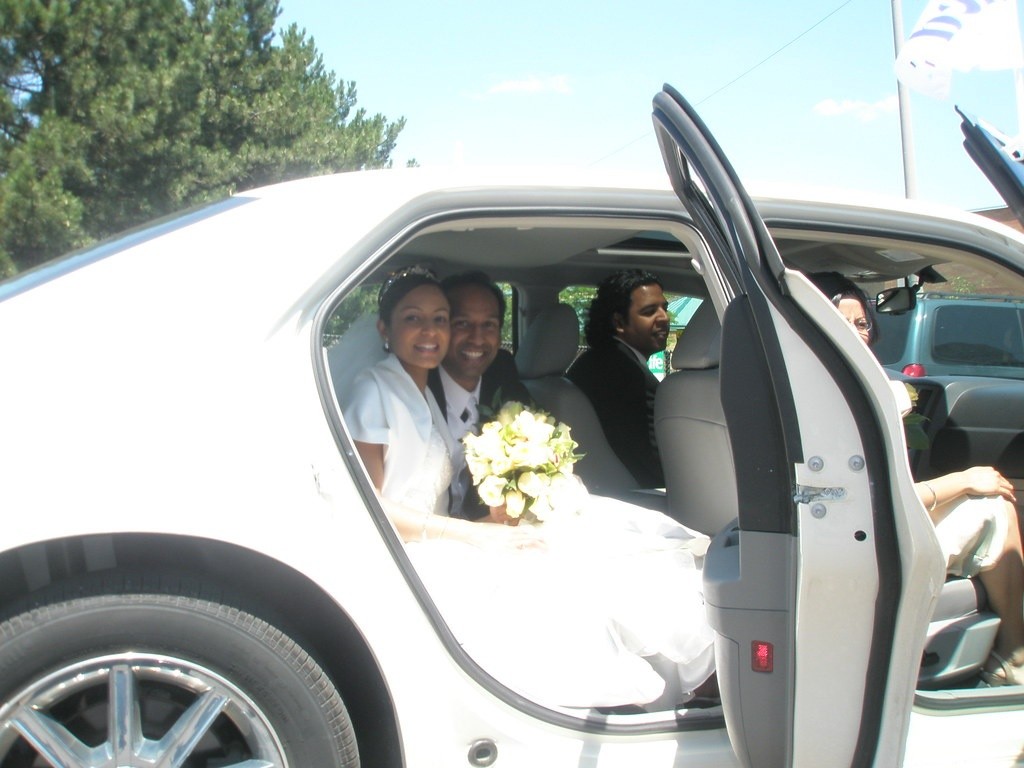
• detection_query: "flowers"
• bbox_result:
[888,380,931,455]
[457,399,590,524]
[429,452,455,492]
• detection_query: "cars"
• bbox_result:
[0,79,1024,768]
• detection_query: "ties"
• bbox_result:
[465,396,481,436]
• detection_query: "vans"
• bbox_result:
[864,273,1024,387]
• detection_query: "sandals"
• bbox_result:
[980,650,1024,687]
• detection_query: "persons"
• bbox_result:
[809,272,1024,687]
[327,266,716,718]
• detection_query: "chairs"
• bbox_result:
[515,304,667,515]
[653,294,739,537]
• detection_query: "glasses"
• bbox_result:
[853,317,873,332]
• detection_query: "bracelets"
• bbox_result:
[921,481,937,511]
[423,514,432,540]
[438,516,449,539]
